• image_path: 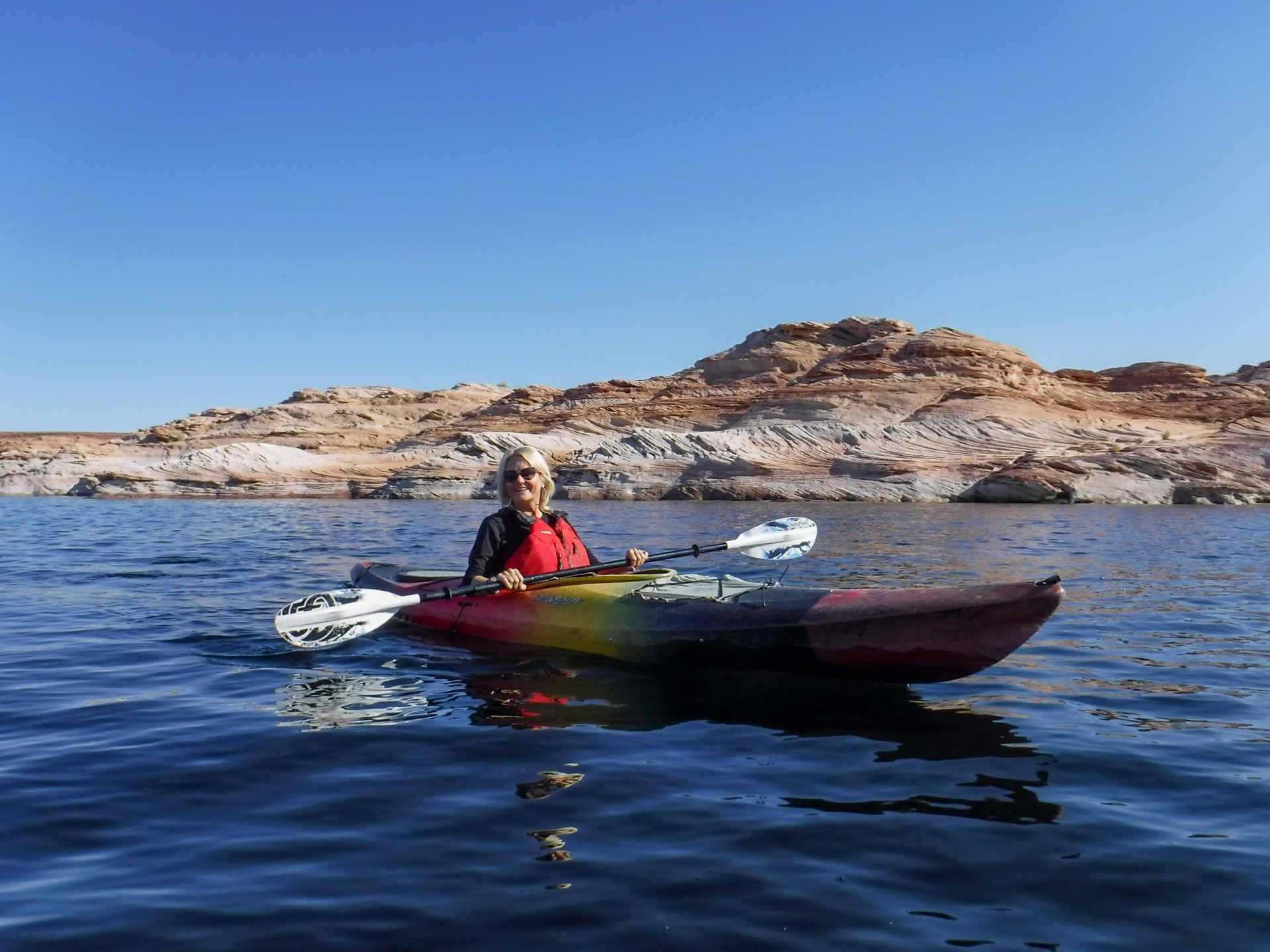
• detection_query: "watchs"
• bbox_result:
[487,575,500,595]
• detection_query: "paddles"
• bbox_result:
[274,516,819,648]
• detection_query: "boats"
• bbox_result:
[349,558,1064,686]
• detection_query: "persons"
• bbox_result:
[459,445,648,596]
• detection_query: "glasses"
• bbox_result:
[503,467,539,483]
